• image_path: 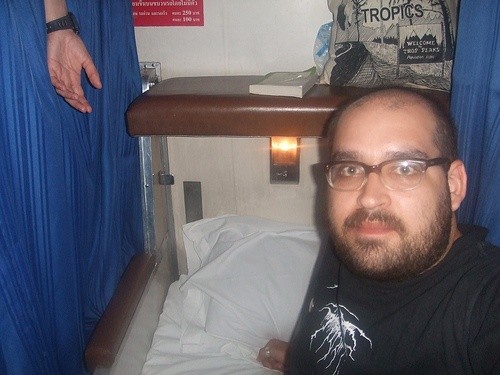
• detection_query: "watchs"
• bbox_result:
[45,11,82,35]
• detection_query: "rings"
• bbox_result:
[264,347,270,358]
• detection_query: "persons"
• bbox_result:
[258,84,500,375]
[44,0,102,113]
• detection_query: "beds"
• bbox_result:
[84,215,328,375]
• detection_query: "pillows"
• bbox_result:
[177,213,330,348]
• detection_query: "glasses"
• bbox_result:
[323,155,451,191]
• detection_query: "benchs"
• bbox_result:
[125,74,449,257]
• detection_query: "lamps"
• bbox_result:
[270,136,301,185]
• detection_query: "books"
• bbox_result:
[248,65,318,99]
